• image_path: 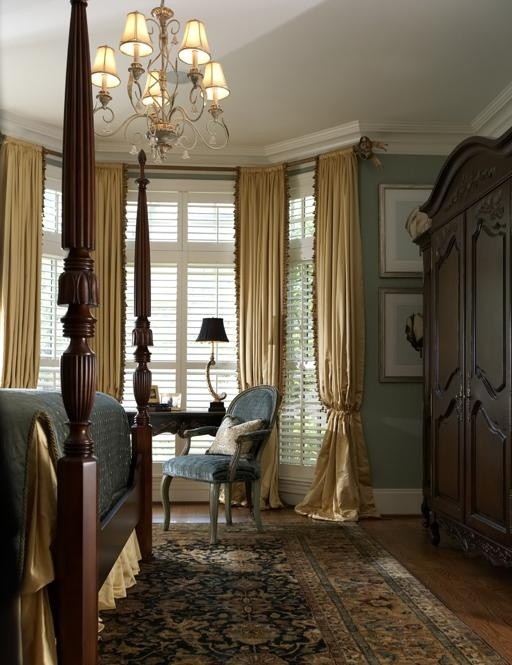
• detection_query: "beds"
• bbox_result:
[0,0,155,665]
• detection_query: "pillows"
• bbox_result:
[207,413,263,459]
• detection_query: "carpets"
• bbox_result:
[95,518,511,664]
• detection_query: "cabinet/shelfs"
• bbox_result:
[412,127,512,570]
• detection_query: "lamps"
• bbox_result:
[89,0,234,162]
[196,318,233,410]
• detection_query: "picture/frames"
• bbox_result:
[148,385,159,405]
[377,183,434,384]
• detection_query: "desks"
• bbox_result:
[121,410,234,525]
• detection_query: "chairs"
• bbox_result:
[161,384,283,546]
